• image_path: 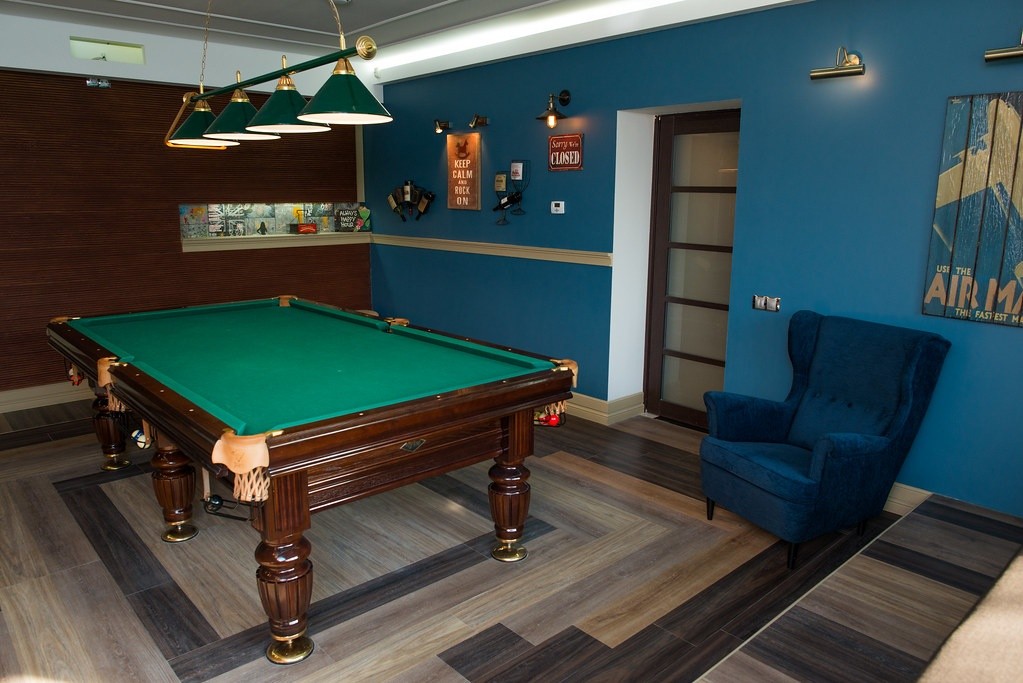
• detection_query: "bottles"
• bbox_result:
[387,193,407,223]
[415,191,435,221]
[404,181,414,215]
[493,192,522,212]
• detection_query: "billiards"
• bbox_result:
[206,494,224,512]
[131,430,151,449]
[533,411,560,426]
[69,368,82,385]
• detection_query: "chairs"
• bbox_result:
[700,310,950,576]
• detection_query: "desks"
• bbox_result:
[42,293,578,666]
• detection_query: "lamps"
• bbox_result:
[469,114,490,128]
[536,91,571,129]
[435,120,452,133]
[156,0,393,149]
[810,46,865,80]
[985,32,1023,61]
